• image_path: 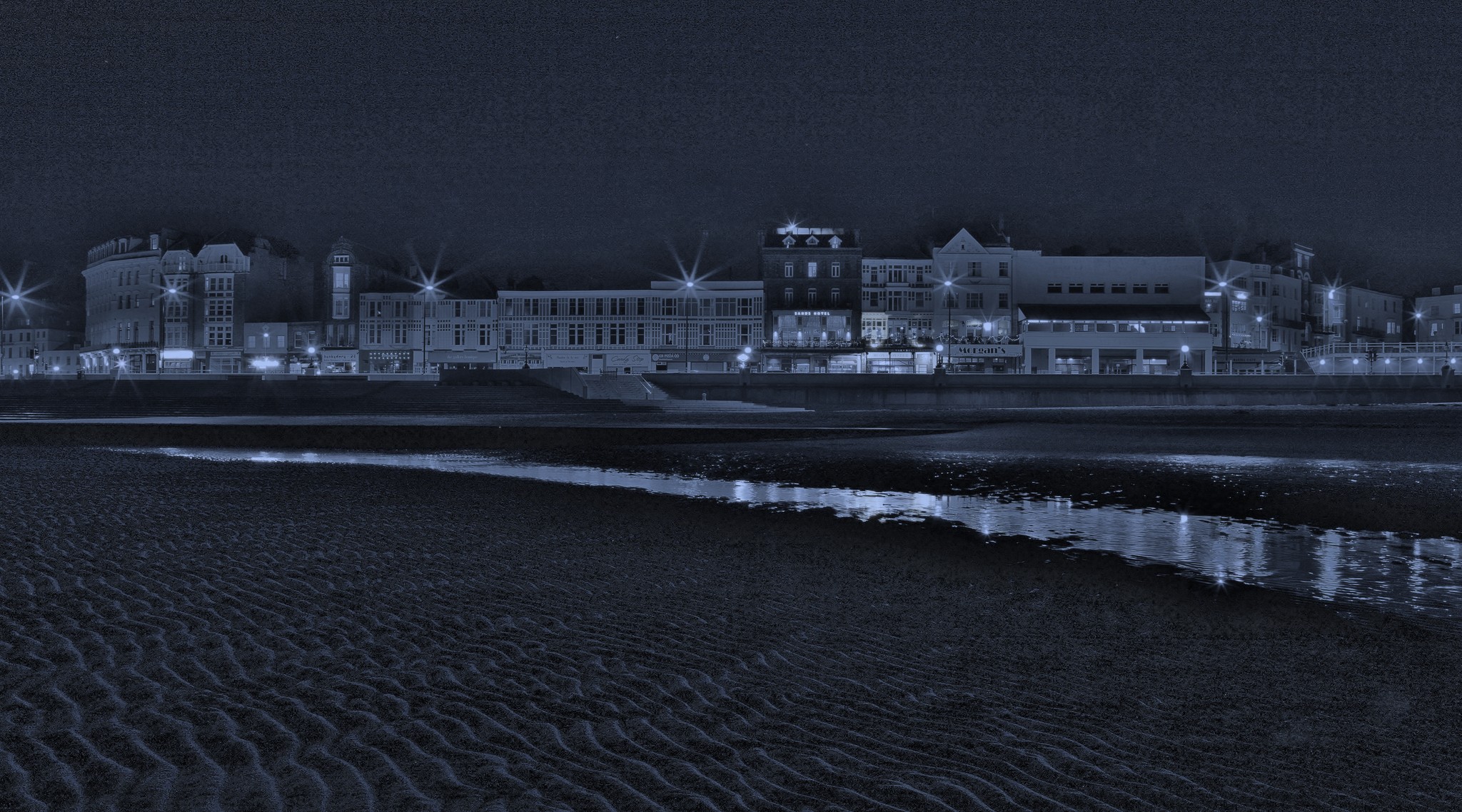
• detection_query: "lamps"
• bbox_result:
[962,321,965,327]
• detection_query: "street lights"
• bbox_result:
[423,283,433,373]
[1416,313,1421,374]
[1181,345,1191,369]
[522,344,530,369]
[744,345,751,367]
[1,295,19,373]
[935,345,944,368]
[1256,316,1262,352]
[113,348,120,368]
[1219,280,1228,374]
[308,347,316,368]
[262,333,269,373]
[684,278,694,373]
[1441,341,1452,369]
[159,273,176,373]
[900,326,904,347]
[944,279,952,373]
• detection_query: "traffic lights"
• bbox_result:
[1373,350,1378,361]
[1365,350,1370,361]
[34,349,39,359]
